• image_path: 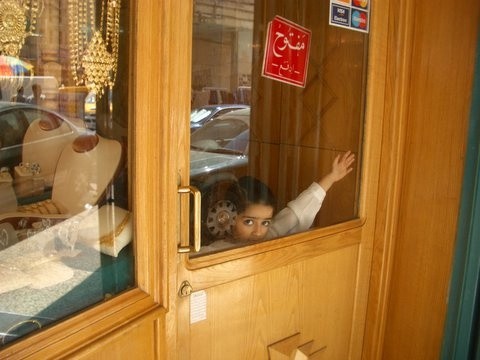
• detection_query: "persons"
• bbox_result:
[8,86,28,103]
[26,84,47,109]
[189,149,357,260]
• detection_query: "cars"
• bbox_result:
[86,87,114,129]
[0,100,97,206]
[190,104,251,134]
[189,109,250,244]
